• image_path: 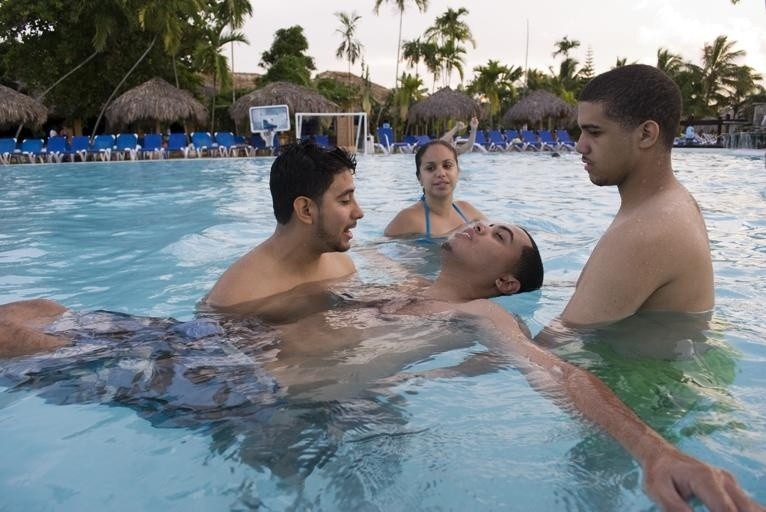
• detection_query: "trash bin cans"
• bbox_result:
[367,135,374,153]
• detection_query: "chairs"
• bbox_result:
[376,125,577,151]
[0,132,281,165]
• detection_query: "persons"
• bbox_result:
[198,139,367,306]
[1,220,766,511]
[386,61,750,387]
[438,116,480,154]
[384,141,492,237]
